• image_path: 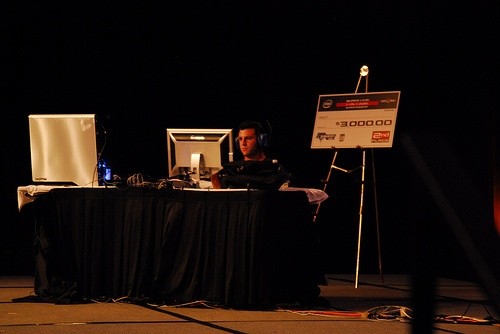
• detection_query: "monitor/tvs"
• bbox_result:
[29,113,97,185]
[166,127,234,189]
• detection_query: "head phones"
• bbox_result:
[235,122,270,149]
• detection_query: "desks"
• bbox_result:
[17,183,329,310]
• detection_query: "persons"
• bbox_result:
[225,122,292,189]
